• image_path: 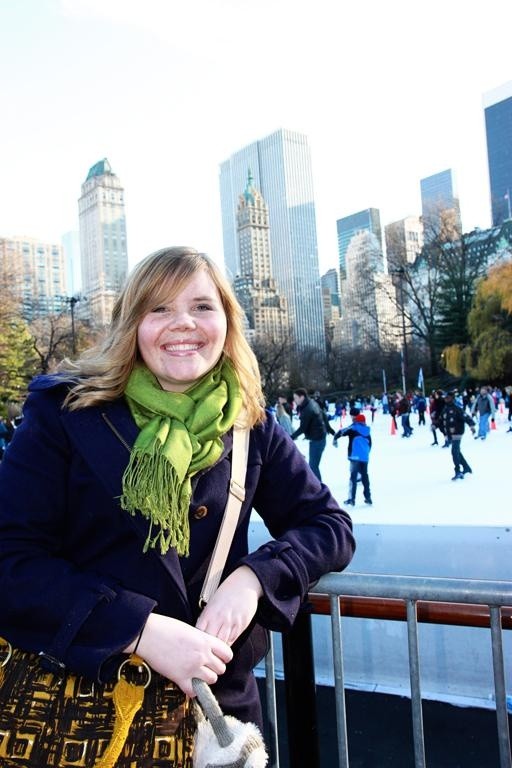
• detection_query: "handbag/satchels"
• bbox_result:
[1,637,202,767]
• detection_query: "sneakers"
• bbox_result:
[364,499,372,504]
[343,499,355,506]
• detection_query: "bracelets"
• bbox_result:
[132,611,147,657]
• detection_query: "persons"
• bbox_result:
[1,246,356,768]
[275,387,511,506]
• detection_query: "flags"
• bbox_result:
[401,346,406,377]
[416,367,424,389]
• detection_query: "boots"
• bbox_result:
[451,466,464,480]
[462,465,472,474]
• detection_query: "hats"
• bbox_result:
[355,414,366,422]
